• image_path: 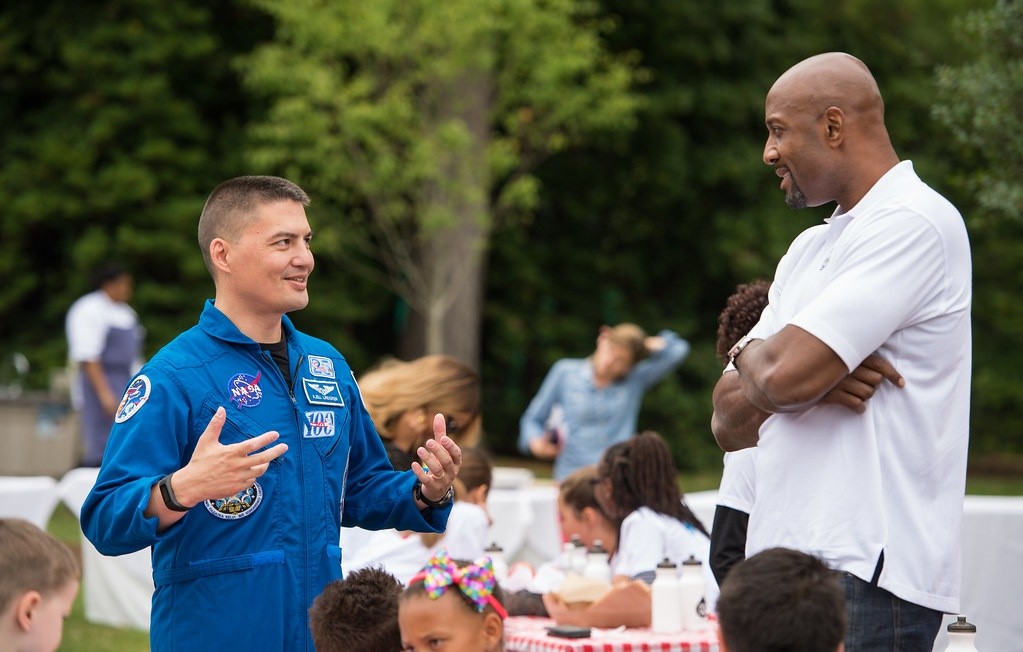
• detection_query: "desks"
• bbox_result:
[0,465,154,632]
[501,615,720,652]
[684,491,1023,652]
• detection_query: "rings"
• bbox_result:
[433,472,445,480]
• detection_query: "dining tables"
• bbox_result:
[483,488,561,563]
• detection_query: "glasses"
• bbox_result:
[445,415,458,436]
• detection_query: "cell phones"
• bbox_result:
[545,626,591,638]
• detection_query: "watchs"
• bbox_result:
[415,480,454,508]
[159,474,192,512]
[727,335,763,371]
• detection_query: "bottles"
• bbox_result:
[484,543,507,585]
[942,615,981,652]
[564,535,611,604]
[650,555,708,637]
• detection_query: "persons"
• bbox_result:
[709,52,969,652]
[81,176,462,652]
[309,567,405,652]
[0,518,80,652]
[398,547,504,652]
[509,429,719,629]
[356,355,493,579]
[708,279,774,588]
[517,323,692,484]
[64,262,145,465]
[715,548,848,652]
